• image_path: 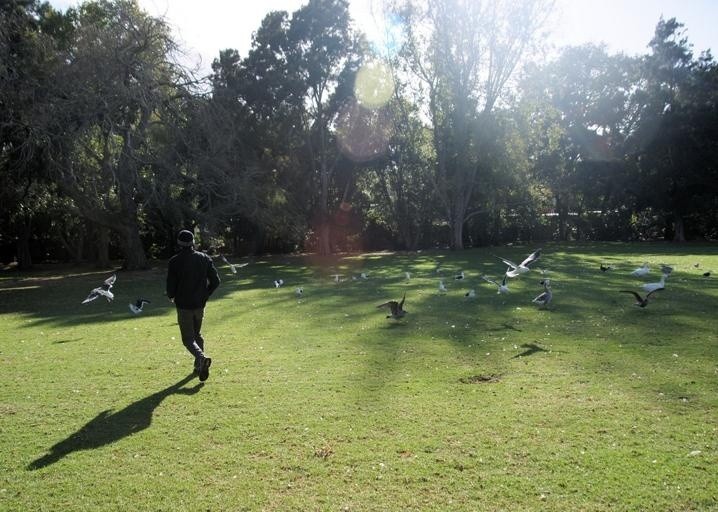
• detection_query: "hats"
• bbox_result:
[177,230,193,247]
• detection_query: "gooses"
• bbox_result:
[631,262,650,279]
[637,275,667,293]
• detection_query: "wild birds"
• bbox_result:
[271,279,284,287]
[438,249,551,309]
[127,297,151,315]
[295,287,304,297]
[660,264,673,277]
[221,255,249,274]
[619,288,665,308]
[376,292,408,320]
[601,264,609,271]
[81,273,117,304]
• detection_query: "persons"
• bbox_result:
[165,229,221,382]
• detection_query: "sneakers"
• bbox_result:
[194,355,210,381]
